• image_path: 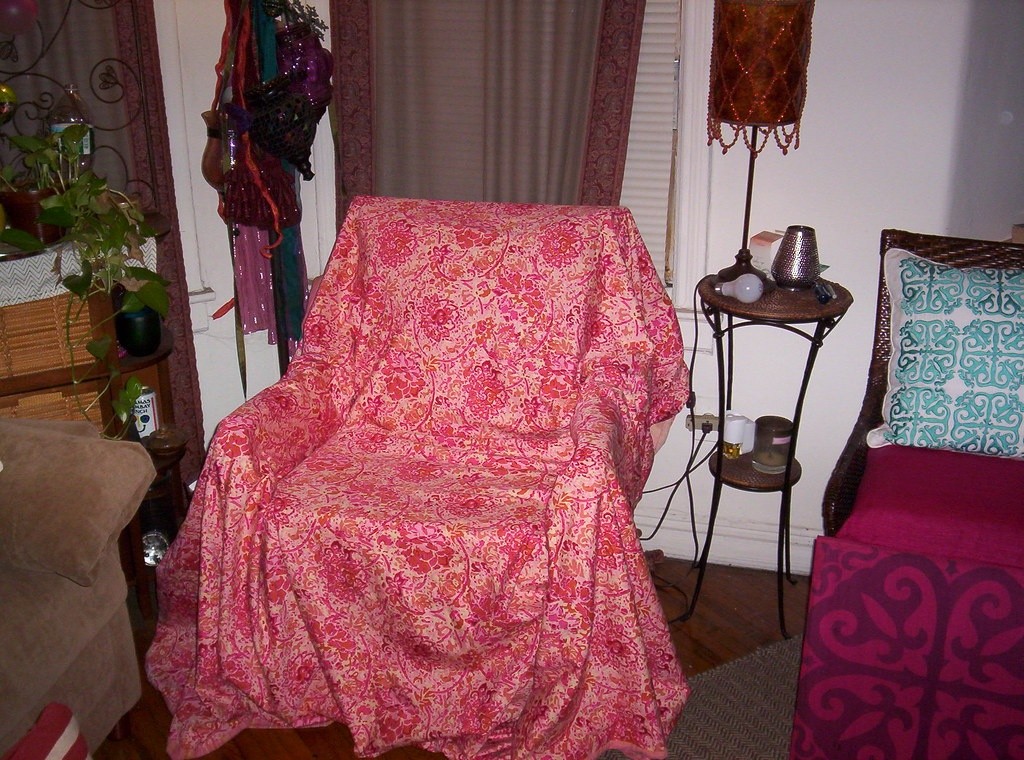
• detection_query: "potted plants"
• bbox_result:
[0,122,173,441]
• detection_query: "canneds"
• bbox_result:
[131,385,159,438]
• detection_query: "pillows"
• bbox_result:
[866,248,1024,463]
[1,425,156,587]
[1,701,93,760]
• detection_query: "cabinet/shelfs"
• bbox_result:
[0,212,187,619]
[667,273,854,642]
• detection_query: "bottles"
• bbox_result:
[771,224,820,292]
[752,415,793,474]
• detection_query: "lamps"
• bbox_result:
[707,0,815,294]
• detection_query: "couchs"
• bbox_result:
[0,418,157,760]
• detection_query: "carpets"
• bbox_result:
[605,634,803,760]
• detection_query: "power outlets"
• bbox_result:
[685,413,719,431]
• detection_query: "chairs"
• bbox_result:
[821,229,1024,569]
[148,194,690,760]
